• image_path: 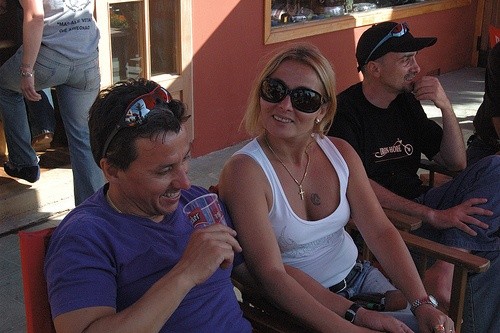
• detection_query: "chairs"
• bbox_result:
[209,180,492,333]
[370,155,460,235]
[19,223,307,333]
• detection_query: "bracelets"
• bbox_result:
[19,68,35,79]
[346,302,368,323]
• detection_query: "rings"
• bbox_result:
[435,324,445,331]
[448,329,453,332]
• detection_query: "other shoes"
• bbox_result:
[33,133,52,151]
[5,161,39,182]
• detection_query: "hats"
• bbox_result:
[356,22,437,72]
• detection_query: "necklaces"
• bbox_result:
[265,133,311,201]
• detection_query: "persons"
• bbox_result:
[1,0,107,213]
[44,78,412,332]
[466,40,500,162]
[218,42,456,333]
[322,21,500,333]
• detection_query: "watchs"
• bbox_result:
[411,294,438,311]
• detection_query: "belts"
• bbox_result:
[328,266,358,294]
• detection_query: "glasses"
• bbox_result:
[260,77,327,113]
[102,86,173,159]
[365,23,410,64]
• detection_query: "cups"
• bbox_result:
[182,194,227,231]
